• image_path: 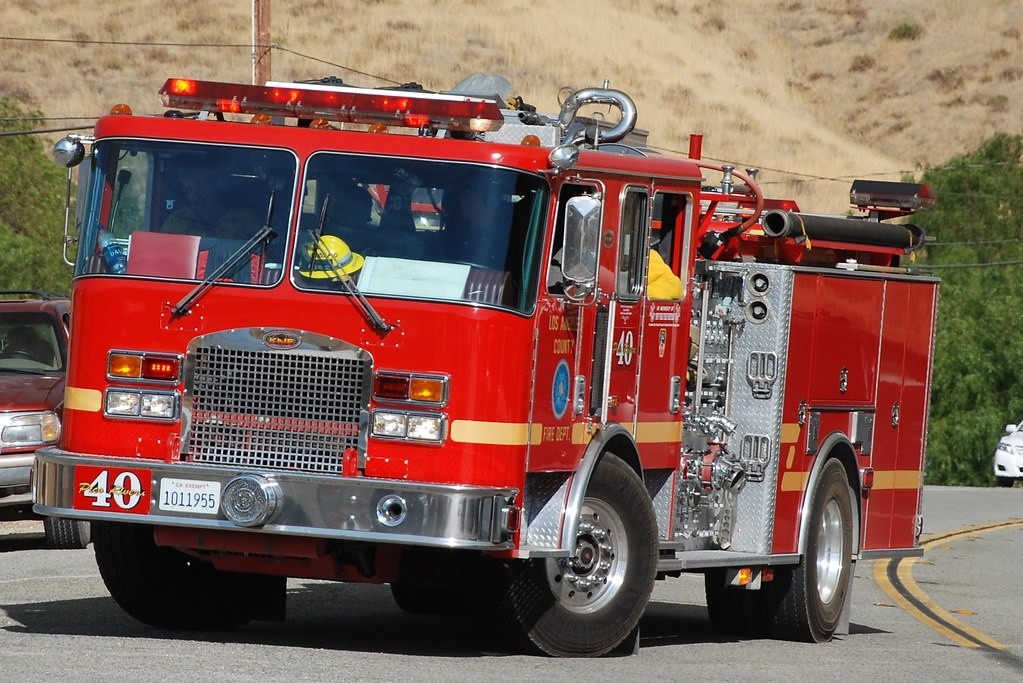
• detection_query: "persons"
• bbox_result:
[473,195,595,295]
[646,248,682,299]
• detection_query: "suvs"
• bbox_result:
[0,289,93,550]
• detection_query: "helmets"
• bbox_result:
[299,234,364,279]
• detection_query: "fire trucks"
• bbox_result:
[28,73,940,662]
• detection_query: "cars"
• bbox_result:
[993,420,1023,488]
